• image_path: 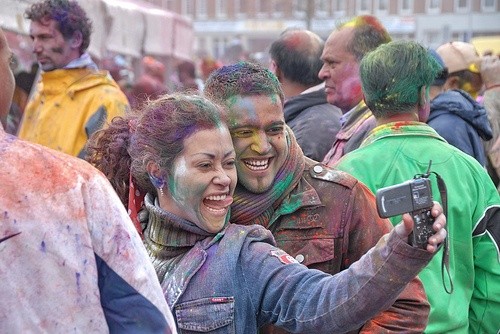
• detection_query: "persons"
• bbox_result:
[126,49,219,112]
[17,1,133,158]
[332,42,500,334]
[204,63,430,334]
[318,15,393,168]
[270,27,344,162]
[425,36,500,185]
[87,94,447,333]
[0,29,176,333]
[12,70,36,119]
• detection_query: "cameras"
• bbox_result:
[375,160,435,251]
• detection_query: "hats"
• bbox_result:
[437,42,487,75]
